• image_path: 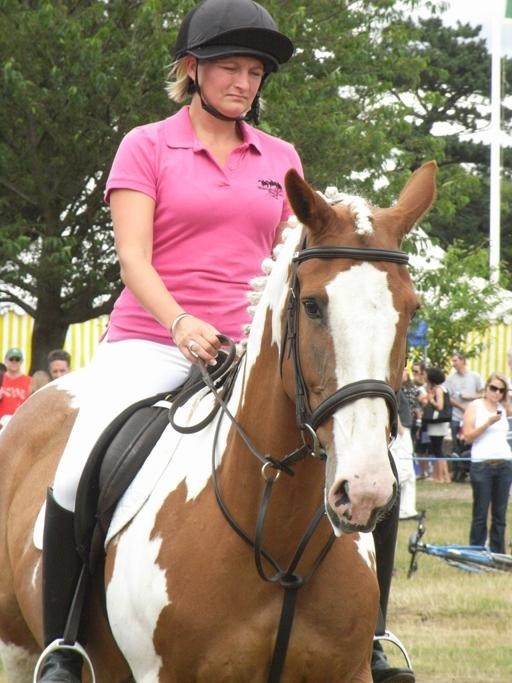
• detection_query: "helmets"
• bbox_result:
[173,0,293,64]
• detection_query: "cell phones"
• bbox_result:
[497,411,502,415]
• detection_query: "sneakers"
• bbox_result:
[399,510,424,520]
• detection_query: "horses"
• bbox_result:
[0,158,439,682]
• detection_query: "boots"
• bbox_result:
[371,498,415,683]
[37,487,87,682]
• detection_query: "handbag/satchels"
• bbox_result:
[423,393,451,422]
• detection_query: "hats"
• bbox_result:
[187,34,281,73]
[8,349,21,359]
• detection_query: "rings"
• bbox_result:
[187,340,197,350]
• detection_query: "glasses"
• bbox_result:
[489,385,504,394]
[10,358,20,362]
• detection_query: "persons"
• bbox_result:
[462,372,512,555]
[38,0,415,683]
[389,353,512,519]
[0,348,71,429]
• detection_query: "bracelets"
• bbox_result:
[171,313,193,340]
